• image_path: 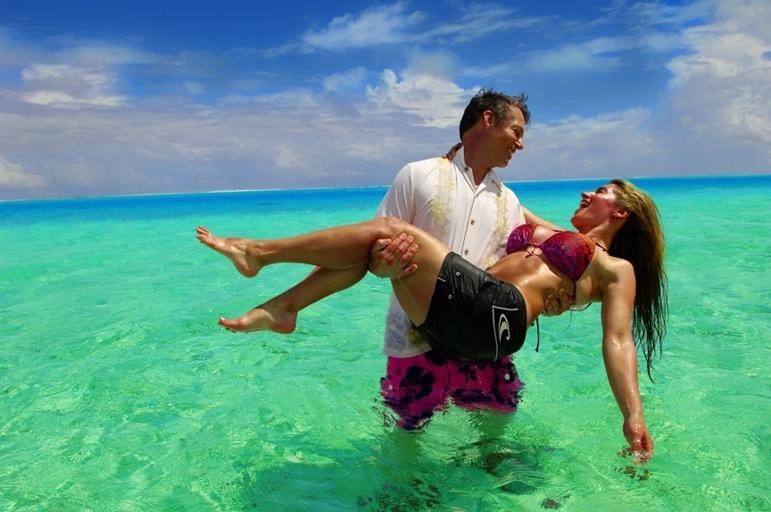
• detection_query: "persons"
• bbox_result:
[367,87,531,429]
[195,179,669,465]
